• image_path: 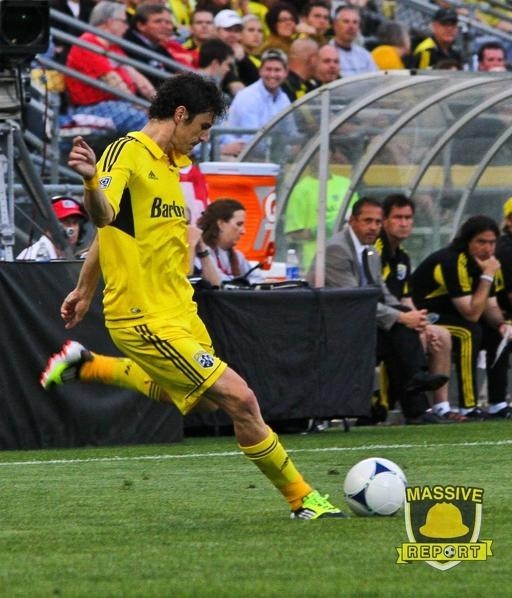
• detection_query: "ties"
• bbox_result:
[362,248,412,312]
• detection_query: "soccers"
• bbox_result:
[344,458,408,517]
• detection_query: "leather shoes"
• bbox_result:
[406,373,449,393]
[408,413,455,426]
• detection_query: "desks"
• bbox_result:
[0,262,381,451]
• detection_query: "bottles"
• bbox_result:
[284,248,300,282]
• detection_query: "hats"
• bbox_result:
[214,9,244,28]
[51,195,88,221]
[433,8,457,22]
[262,47,288,64]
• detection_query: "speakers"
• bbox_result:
[0,0,50,54]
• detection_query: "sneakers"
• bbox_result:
[289,489,345,519]
[441,406,512,422]
[40,338,86,391]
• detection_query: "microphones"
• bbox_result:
[230,262,264,285]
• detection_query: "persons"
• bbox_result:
[16,0,511,520]
